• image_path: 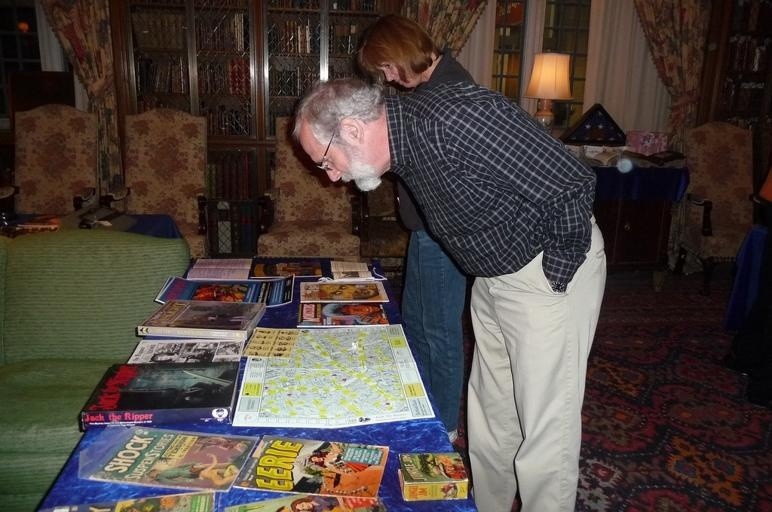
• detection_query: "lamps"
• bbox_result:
[522,53,574,136]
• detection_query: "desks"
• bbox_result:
[36,259,478,512]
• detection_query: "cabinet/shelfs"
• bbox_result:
[594,166,690,283]
[110,0,405,259]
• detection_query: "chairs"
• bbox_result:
[0,104,100,232]
[257,117,360,257]
[99,108,209,261]
[361,172,411,267]
[672,120,766,296]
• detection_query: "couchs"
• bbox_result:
[0,231,191,512]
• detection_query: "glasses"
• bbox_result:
[316,130,337,173]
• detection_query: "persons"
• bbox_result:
[355,14,477,447]
[292,72,609,512]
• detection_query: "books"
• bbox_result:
[36,254,478,512]
